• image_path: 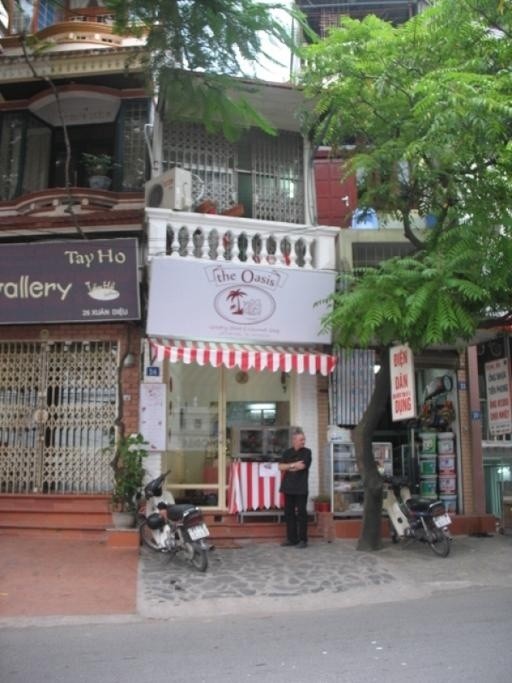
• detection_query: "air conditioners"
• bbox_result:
[143,166,193,212]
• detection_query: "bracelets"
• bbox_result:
[288,462,296,469]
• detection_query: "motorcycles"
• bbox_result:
[134,470,215,572]
[382,476,453,558]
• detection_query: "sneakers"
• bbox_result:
[279,539,308,549]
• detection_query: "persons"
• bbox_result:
[279,429,312,549]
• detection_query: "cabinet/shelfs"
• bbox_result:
[325,441,394,519]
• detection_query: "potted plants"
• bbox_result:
[94,431,151,529]
[78,151,123,190]
